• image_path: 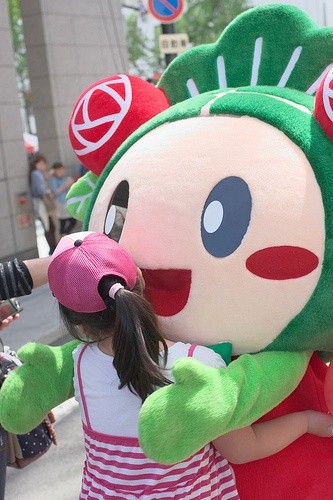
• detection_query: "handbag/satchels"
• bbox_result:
[0,350,57,470]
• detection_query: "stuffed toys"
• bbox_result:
[0,3,333,499]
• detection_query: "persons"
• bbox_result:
[0,255,52,500]
[28,156,77,255]
[48,231,333,500]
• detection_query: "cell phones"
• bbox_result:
[0,298,24,324]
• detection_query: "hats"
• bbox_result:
[49,232,137,313]
